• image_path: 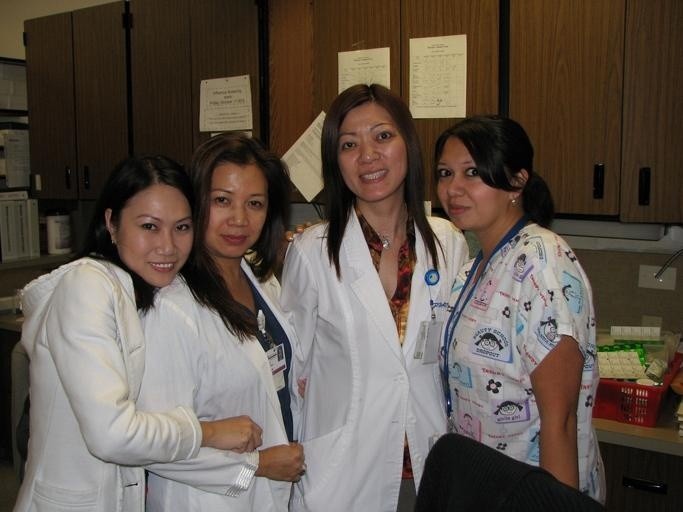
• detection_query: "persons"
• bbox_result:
[278,82,471,511]
[134,133,308,512]
[12,148,323,512]
[433,117,607,509]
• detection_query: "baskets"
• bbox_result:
[592,352,683,428]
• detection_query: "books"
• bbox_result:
[0,190,40,261]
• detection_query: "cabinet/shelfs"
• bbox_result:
[81,21,82,27]
[266,1,500,205]
[128,0,268,167]
[22,0,133,200]
[507,0,682,225]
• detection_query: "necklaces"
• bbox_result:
[371,205,408,250]
